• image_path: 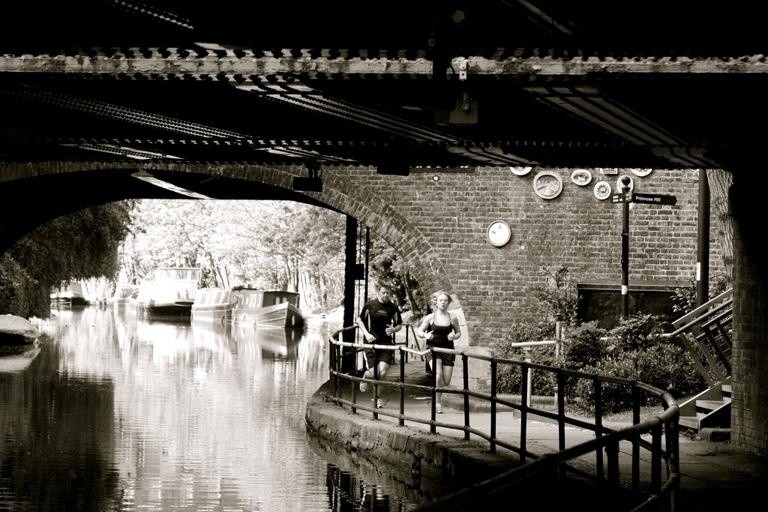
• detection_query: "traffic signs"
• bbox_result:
[609,192,625,203]
[631,192,678,207]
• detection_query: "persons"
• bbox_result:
[415,290,462,413]
[357,282,404,408]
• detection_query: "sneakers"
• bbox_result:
[359,370,370,393]
[436,403,443,413]
[376,398,384,409]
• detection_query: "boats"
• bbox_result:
[47,268,307,335]
[0,312,41,346]
[255,326,300,367]
[0,343,42,375]
